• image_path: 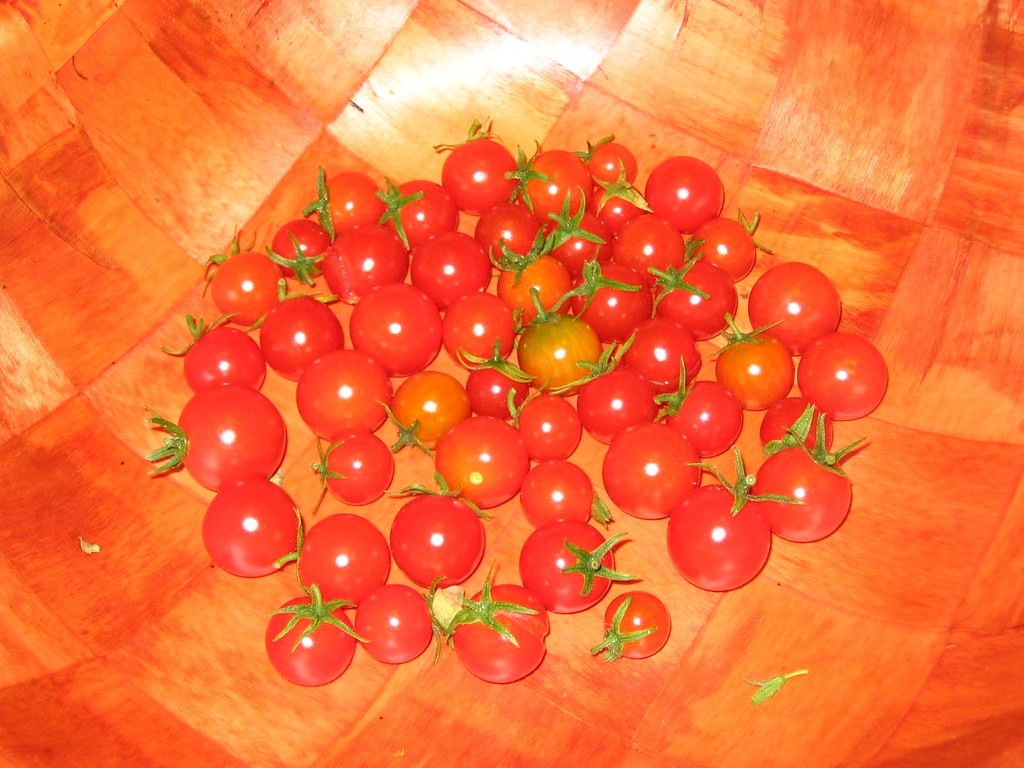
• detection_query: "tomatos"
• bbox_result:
[147,118,891,685]
